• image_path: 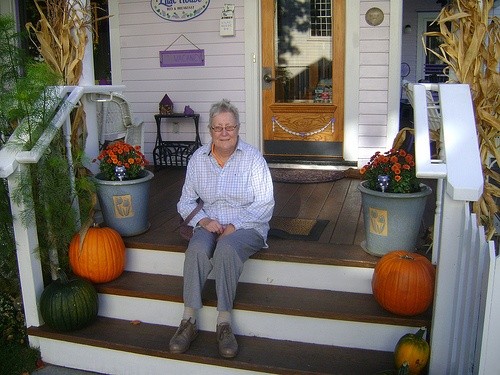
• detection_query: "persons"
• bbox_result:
[169,98,275,358]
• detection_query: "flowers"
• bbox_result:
[358,150,419,193]
[92,142,150,181]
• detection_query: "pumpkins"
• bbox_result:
[394,329,430,375]
[371,250,435,316]
[68,222,126,283]
[39,271,98,332]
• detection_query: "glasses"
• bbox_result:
[210,123,239,132]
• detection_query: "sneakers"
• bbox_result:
[169,319,198,354]
[216,322,238,358]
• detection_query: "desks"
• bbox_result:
[152,113,202,170]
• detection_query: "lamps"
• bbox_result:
[404,24,411,34]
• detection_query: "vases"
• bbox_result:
[358,179,432,256]
[92,170,154,238]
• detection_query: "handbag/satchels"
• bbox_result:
[176,199,204,240]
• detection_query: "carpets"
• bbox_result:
[270,168,346,184]
[267,216,330,241]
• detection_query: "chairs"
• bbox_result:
[402,84,440,158]
[84,93,144,157]
